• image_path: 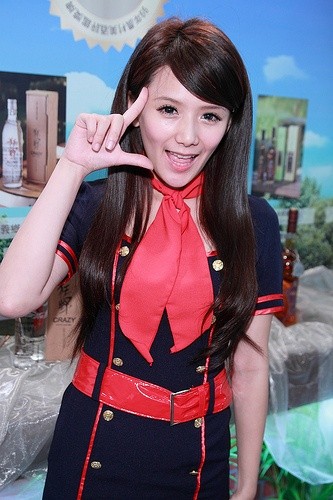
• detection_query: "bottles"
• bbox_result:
[257,130,266,184]
[1,99,23,189]
[14,298,46,369]
[277,207,301,326]
[266,128,277,183]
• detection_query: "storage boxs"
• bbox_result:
[25,90,58,186]
[45,271,83,363]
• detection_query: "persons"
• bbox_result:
[0,16,288,500]
